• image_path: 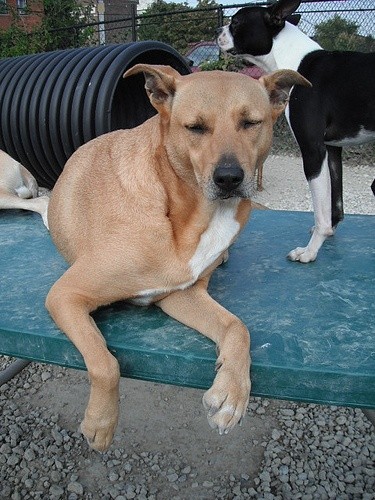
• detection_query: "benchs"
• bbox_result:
[0,210,375,423]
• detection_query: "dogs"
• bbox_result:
[47,62,312,456]
[214,0,375,264]
[0,149,51,231]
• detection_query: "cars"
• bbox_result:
[184,39,275,83]
